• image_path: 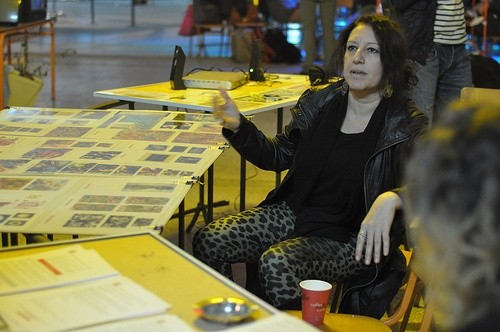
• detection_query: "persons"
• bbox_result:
[267,0,338,76]
[389,0,474,128]
[191,14,429,320]
[402,98,500,332]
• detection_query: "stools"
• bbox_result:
[193,22,263,62]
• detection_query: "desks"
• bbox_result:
[0,19,328,332]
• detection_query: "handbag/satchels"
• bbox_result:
[179,0,305,68]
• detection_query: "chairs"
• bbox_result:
[279,217,435,332]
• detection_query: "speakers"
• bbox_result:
[170,45,187,90]
[248,39,266,81]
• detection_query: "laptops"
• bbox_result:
[0,0,48,27]
